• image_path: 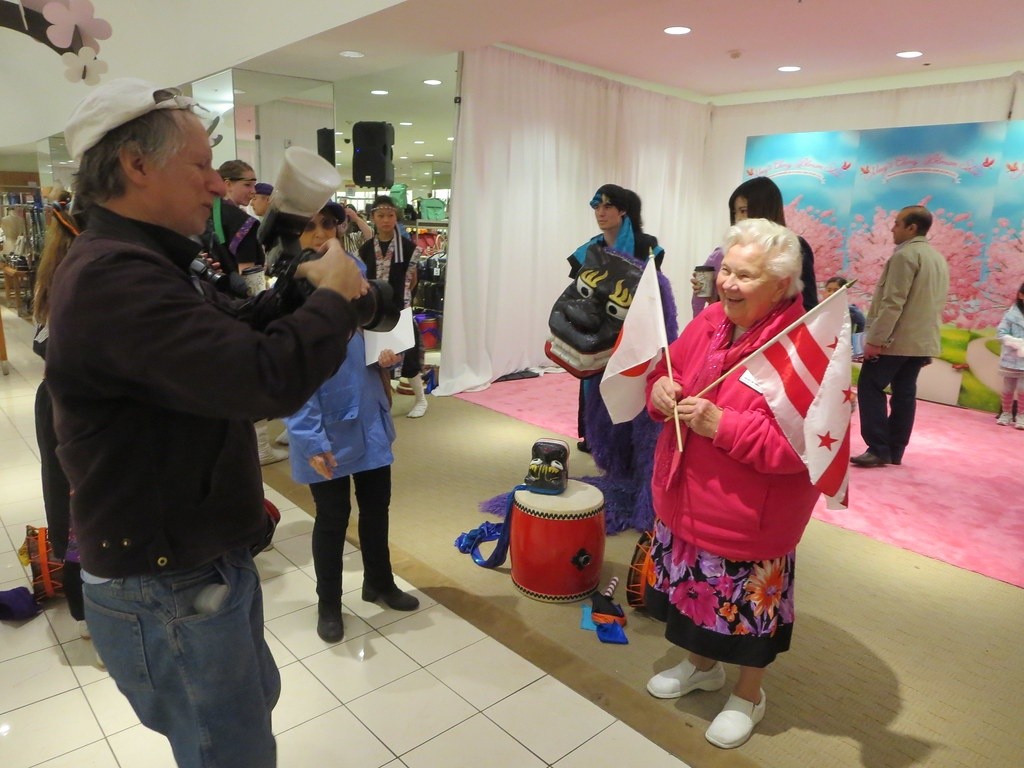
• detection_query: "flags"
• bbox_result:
[742,285,852,510]
[599,254,668,424]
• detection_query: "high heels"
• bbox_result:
[361,579,419,611]
[317,599,344,642]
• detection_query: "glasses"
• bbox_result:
[304,216,338,231]
[857,355,880,363]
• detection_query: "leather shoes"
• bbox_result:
[850,452,892,465]
[892,456,901,464]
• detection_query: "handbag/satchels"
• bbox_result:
[420,198,445,220]
[412,280,444,311]
[417,233,448,282]
[389,183,408,210]
[411,232,441,255]
[403,204,416,221]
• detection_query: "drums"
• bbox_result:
[509,479,608,604]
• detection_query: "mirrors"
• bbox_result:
[192,69,334,188]
[36,137,79,191]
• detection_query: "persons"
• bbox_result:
[202,158,427,422]
[645,176,821,749]
[275,198,420,645]
[35,208,103,668]
[45,78,366,768]
[568,184,664,453]
[995,282,1024,429]
[849,206,949,466]
[825,276,866,333]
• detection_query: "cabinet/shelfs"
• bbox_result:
[0,172,41,288]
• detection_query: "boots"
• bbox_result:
[253,418,288,465]
[407,371,428,417]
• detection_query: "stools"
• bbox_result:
[3,266,34,317]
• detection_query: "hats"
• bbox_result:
[326,198,346,225]
[66,77,210,215]
[254,183,274,195]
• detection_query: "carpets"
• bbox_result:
[452,368,1024,587]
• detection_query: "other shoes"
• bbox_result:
[577,442,590,452]
[78,619,91,640]
[275,428,289,444]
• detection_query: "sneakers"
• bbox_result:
[646,657,726,698]
[996,411,1013,426]
[704,687,766,748]
[1014,414,1024,429]
